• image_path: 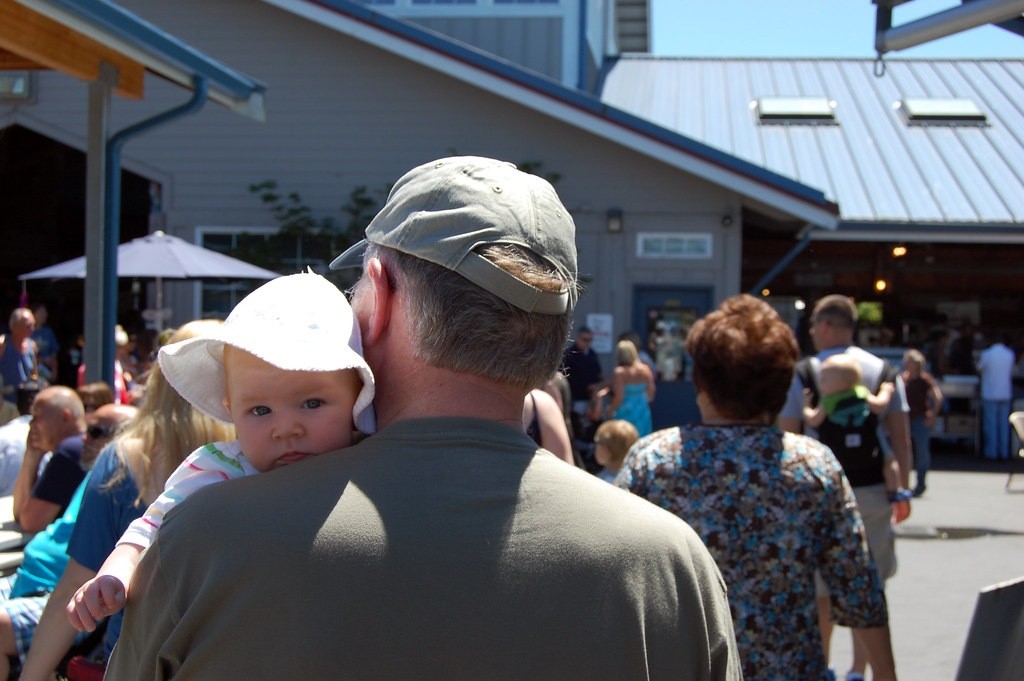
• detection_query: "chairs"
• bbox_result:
[1004,409,1024,490]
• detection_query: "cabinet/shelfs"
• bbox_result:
[928,380,984,458]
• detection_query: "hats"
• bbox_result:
[157,269,377,435]
[331,154,580,316]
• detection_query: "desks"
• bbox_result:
[0,495,34,577]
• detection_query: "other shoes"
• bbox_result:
[913,485,926,497]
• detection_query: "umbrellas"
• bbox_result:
[18,230,284,333]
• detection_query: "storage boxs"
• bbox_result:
[934,413,974,433]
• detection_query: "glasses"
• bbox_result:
[86,425,114,439]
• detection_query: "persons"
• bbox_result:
[610,296,895,681]
[0,305,238,681]
[67,260,377,633]
[521,323,656,485]
[103,155,745,681]
[637,316,703,433]
[926,321,1015,464]
[780,294,942,681]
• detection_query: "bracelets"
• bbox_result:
[888,487,913,504]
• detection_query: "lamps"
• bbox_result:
[608,205,623,234]
